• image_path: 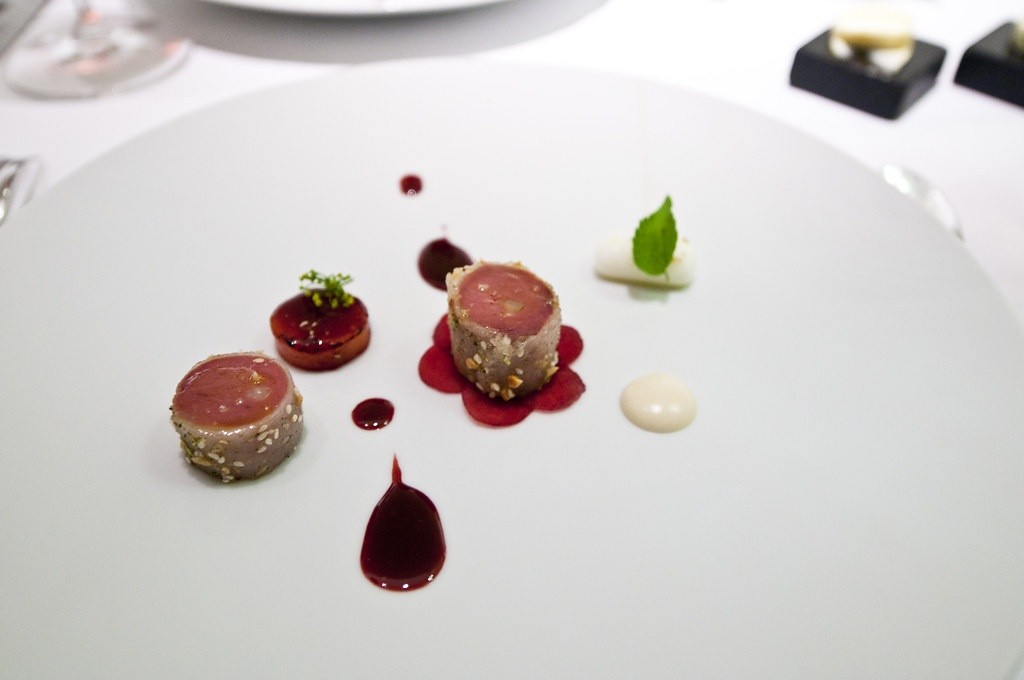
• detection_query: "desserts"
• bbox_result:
[445,261,562,403]
[168,351,303,482]
[270,289,370,371]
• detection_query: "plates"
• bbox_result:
[2,57,1024,680]
[205,0,518,14]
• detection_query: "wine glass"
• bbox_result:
[2,0,193,101]
